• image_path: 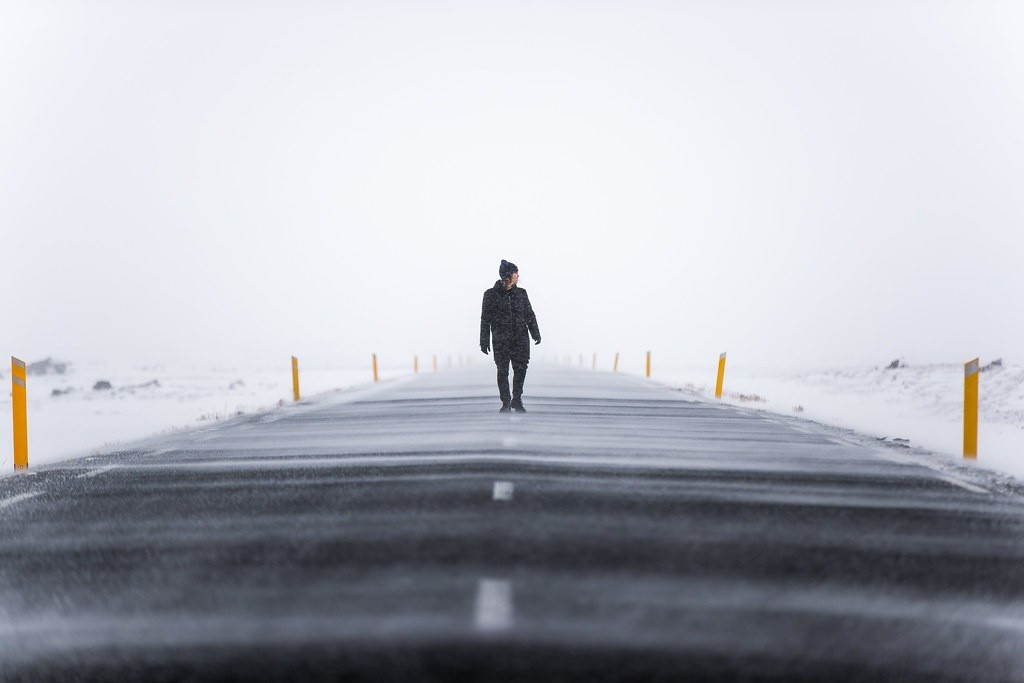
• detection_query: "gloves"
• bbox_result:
[534,337,540,345]
[481,345,491,354]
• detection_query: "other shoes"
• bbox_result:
[501,400,510,413]
[510,397,526,412]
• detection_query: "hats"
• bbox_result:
[499,259,518,278]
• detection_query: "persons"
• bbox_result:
[480,260,541,413]
